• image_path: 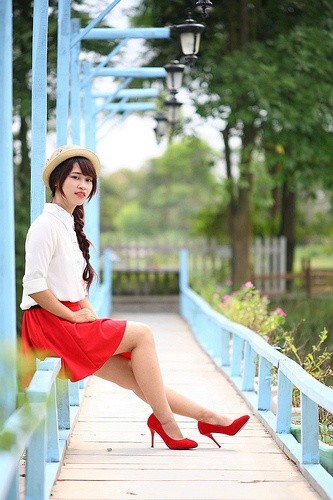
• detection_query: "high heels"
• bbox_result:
[147,413,198,449]
[197,414,250,448]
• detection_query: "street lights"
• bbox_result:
[83,90,183,309]
[70,16,205,146]
[30,0,214,226]
[83,95,182,288]
[164,61,184,95]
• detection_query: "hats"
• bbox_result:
[42,144,101,189]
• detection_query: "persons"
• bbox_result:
[20,146,249,451]
